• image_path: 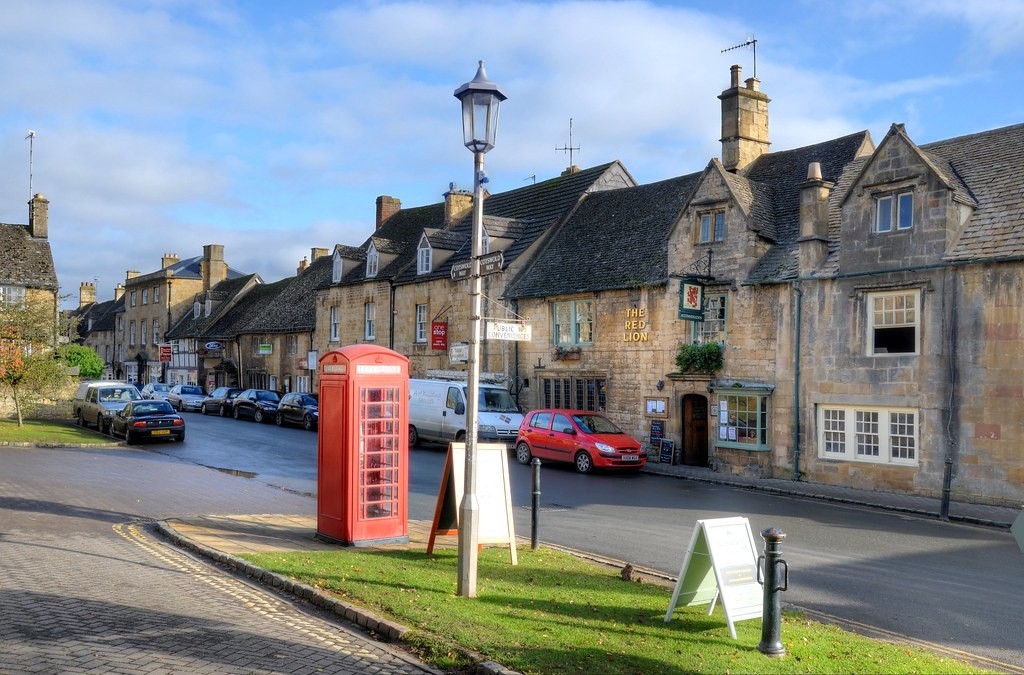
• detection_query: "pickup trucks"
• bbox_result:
[73,382,144,431]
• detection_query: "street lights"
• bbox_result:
[454,59,507,595]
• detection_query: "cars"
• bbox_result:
[516,410,647,471]
[233,389,281,422]
[168,385,208,412]
[141,383,171,401]
[109,401,185,444]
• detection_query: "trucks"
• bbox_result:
[408,380,524,452]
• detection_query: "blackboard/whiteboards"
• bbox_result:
[650,419,666,447]
[659,439,675,466]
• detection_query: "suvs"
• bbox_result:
[277,392,318,429]
[201,387,246,416]
